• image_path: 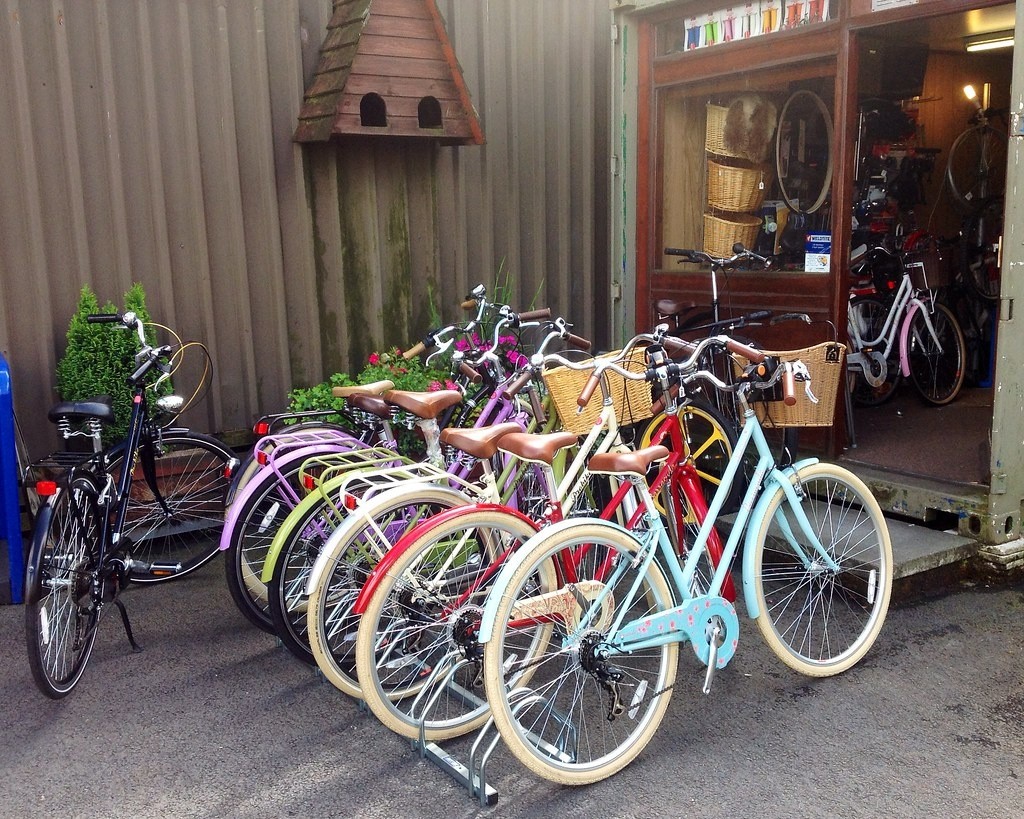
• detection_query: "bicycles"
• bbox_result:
[14,312,244,699]
[219,78,1014,791]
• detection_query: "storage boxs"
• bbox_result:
[704,102,772,160]
[702,212,763,261]
[706,156,774,212]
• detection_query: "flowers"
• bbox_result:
[368,330,529,393]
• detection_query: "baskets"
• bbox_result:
[705,97,774,162]
[537,346,658,437]
[701,210,765,261]
[702,157,776,215]
[730,341,846,431]
